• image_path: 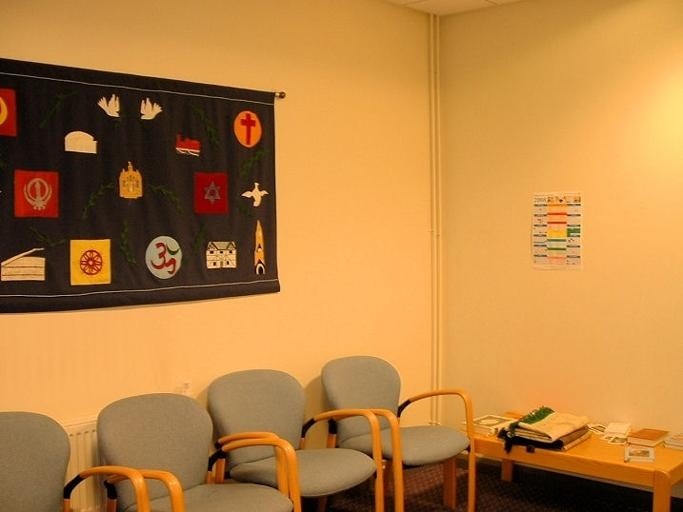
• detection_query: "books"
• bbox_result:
[461,413,518,436]
[664,433,683,451]
[604,421,632,439]
[626,428,669,447]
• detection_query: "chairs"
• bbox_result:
[321,356,478,511]
[97,394,302,511]
[207,368,385,512]
[0,411,151,512]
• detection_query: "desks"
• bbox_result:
[461,413,683,512]
